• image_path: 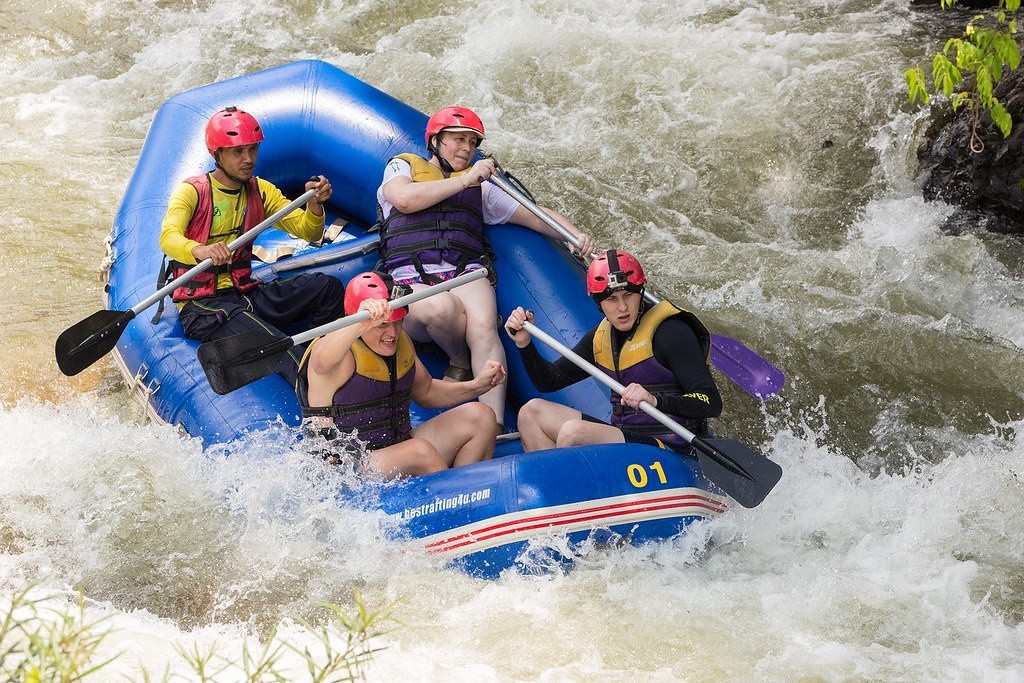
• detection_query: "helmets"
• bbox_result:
[343,271,413,324]
[586,249,647,296]
[425,106,487,150]
[205,106,265,151]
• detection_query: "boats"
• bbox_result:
[107,58,730,577]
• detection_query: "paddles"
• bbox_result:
[508,310,784,510]
[53,174,327,377]
[195,254,498,397]
[477,153,786,404]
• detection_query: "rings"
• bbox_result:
[503,373,506,377]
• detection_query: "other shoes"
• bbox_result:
[440,360,472,383]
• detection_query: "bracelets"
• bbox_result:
[460,172,472,190]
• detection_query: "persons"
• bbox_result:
[378,106,598,443]
[504,249,723,462]
[297,271,508,484]
[159,106,346,381]
